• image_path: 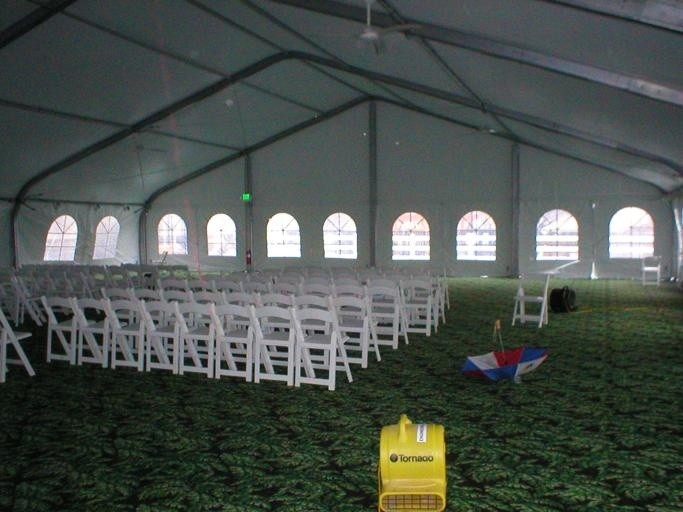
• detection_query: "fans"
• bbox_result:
[313,0,423,57]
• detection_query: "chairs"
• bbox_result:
[512,270,560,328]
[0,262,450,391]
[641,254,662,287]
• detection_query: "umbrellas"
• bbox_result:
[461,319,548,381]
